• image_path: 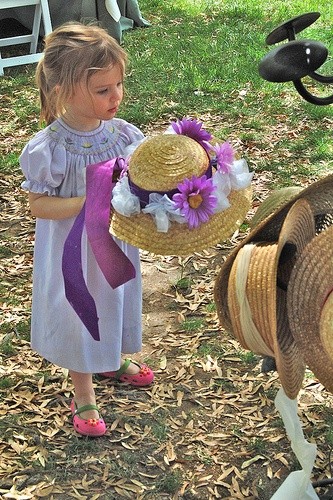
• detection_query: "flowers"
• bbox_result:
[113,117,251,232]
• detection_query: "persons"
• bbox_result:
[19,24,153,436]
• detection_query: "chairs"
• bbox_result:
[0,0,52,77]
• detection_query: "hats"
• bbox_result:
[227,197,306,399]
[97,116,253,257]
[286,224,333,394]
[214,173,332,338]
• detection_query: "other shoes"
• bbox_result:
[98,358,154,386]
[71,398,106,436]
